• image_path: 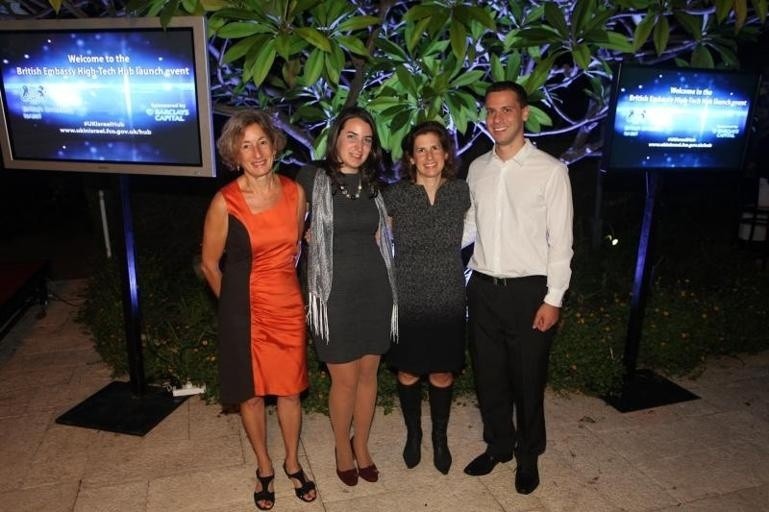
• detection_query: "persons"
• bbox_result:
[201,109,317,509]
[461,81,574,495]
[295,107,398,485]
[381,120,471,475]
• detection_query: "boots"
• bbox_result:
[397,379,422,469]
[428,383,453,475]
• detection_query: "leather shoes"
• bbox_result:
[464,450,513,476]
[515,450,539,494]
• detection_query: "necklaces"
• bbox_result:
[339,173,362,201]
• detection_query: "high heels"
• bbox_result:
[350,436,378,482]
[335,449,358,486]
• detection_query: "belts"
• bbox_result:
[472,270,548,288]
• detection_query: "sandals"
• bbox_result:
[254,467,275,510]
[283,459,317,502]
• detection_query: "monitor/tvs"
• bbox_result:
[1,14,221,180]
[601,60,753,174]
